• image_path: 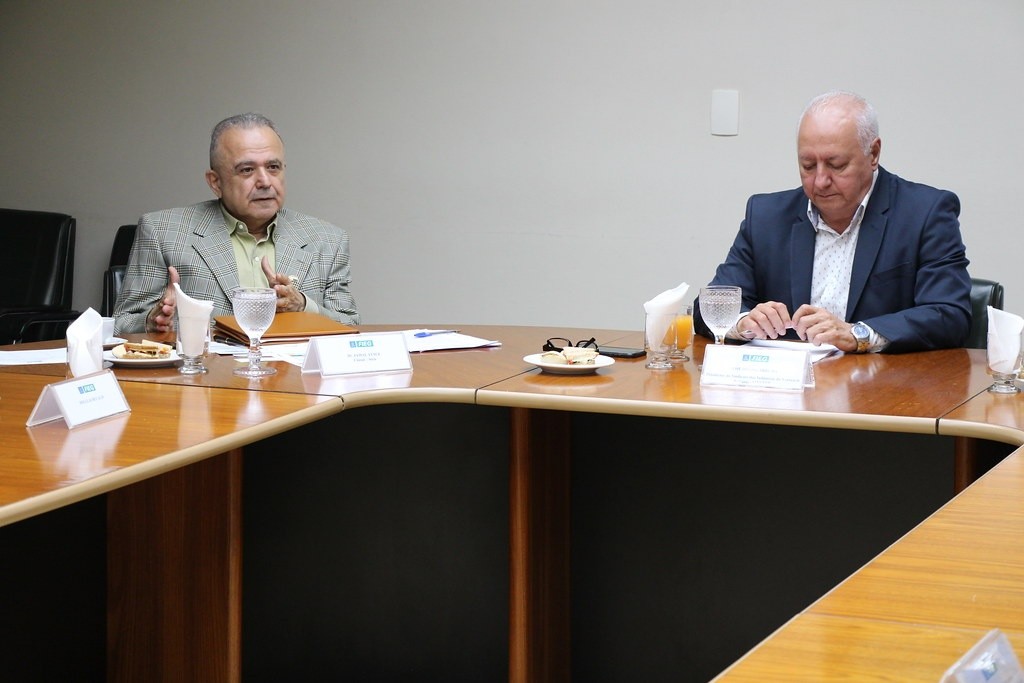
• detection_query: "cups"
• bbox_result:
[101,317,115,344]
[65,347,103,379]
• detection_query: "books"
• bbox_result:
[213,311,359,347]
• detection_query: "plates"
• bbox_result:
[103,350,183,366]
[102,337,128,350]
[1013,369,1024,382]
[523,353,615,375]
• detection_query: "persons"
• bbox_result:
[693,91,975,355]
[112,112,361,337]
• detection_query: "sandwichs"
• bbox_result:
[540,346,600,367]
[120,338,173,360]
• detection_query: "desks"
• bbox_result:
[1,325,1024,683]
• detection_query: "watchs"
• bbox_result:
[849,323,870,354]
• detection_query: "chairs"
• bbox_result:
[0,209,138,341]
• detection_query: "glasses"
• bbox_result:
[543,337,599,353]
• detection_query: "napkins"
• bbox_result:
[986,305,1024,373]
[645,281,692,350]
[173,282,213,357]
[66,306,105,378]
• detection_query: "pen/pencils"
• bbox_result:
[739,323,793,334]
[216,339,236,345]
[414,330,461,337]
[213,335,245,346]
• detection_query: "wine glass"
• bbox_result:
[176,317,211,375]
[645,313,676,368]
[660,306,692,363]
[698,286,742,370]
[232,287,277,376]
[986,332,1024,394]
